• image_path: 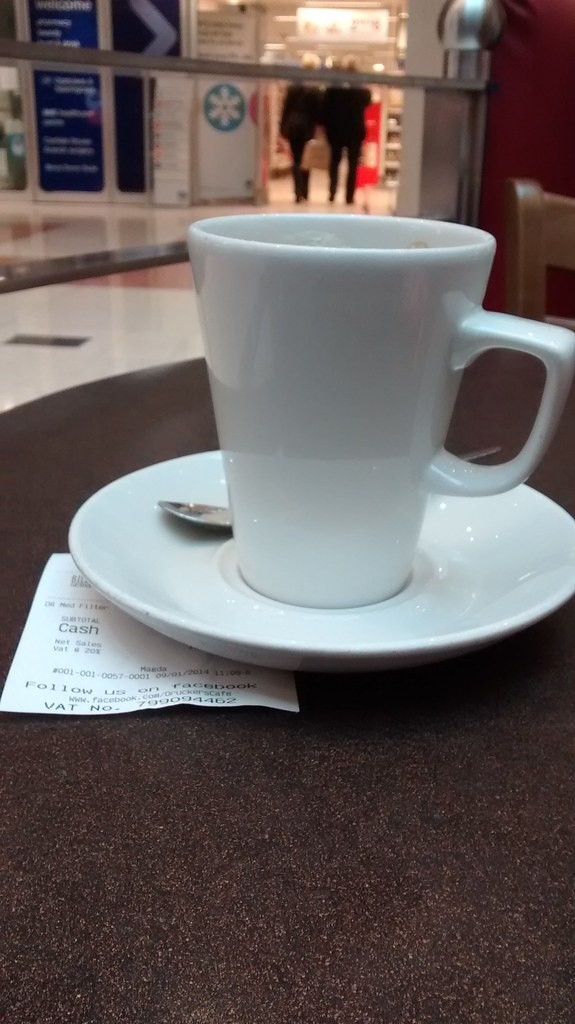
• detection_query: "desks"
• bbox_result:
[0,331,575,1024]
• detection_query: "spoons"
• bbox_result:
[158,428,510,540]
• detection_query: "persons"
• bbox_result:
[278,79,322,202]
[319,81,371,203]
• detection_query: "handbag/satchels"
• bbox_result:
[300,128,331,170]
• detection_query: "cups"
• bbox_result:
[187,214,575,606]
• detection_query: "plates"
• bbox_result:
[68,447,575,668]
